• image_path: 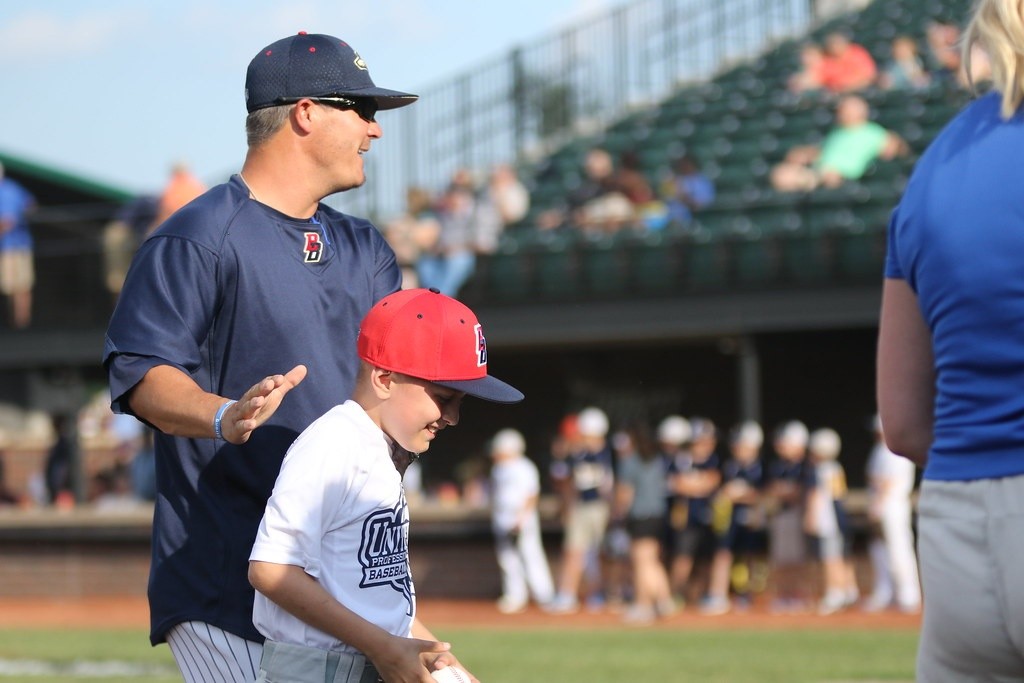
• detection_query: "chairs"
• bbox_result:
[453,0,997,306]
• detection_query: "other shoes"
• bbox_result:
[489,586,922,619]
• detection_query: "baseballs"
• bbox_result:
[430,665,471,683]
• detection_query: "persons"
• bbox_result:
[0,162,35,329]
[534,146,714,235]
[248,288,525,683]
[788,21,992,94]
[404,407,921,618]
[106,163,204,301]
[772,98,908,190]
[0,370,157,508]
[104,32,419,683]
[382,167,527,298]
[876,0,1024,683]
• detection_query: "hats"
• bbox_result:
[776,421,807,446]
[357,286,525,405]
[489,427,526,452]
[244,31,420,110]
[560,415,583,440]
[657,415,692,445]
[729,419,765,446]
[576,408,609,436]
[808,428,846,455]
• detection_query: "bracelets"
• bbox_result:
[214,400,237,442]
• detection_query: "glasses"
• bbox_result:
[278,95,379,121]
